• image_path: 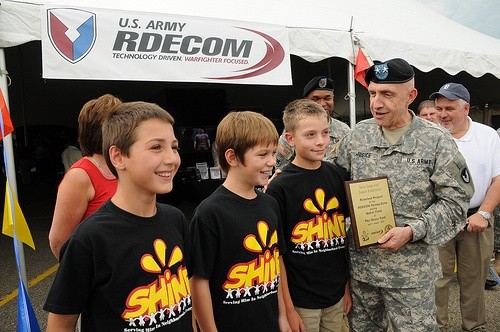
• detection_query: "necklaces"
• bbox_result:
[91,157,115,177]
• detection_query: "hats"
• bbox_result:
[365,59,414,84]
[302,75,335,97]
[429,83,470,102]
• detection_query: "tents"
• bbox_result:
[0,0,500,296]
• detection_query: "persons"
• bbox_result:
[275,76,350,172]
[49,93,123,261]
[43,101,201,332]
[492,128,500,276]
[61,136,81,172]
[429,83,500,332]
[265,100,352,332]
[194,128,210,151]
[417,100,499,288]
[187,111,293,332]
[262,58,475,332]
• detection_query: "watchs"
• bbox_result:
[477,210,492,219]
